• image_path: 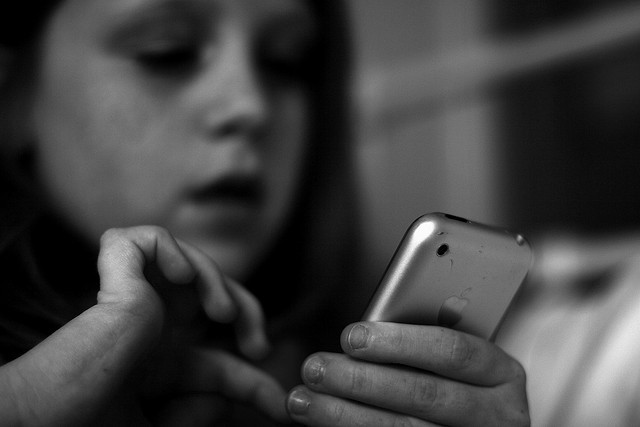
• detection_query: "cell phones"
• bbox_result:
[359,212,534,344]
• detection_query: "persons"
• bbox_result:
[1,1,529,425]
